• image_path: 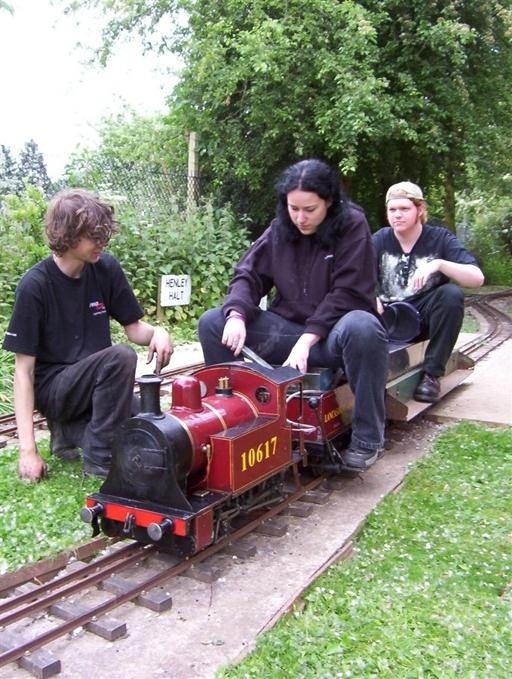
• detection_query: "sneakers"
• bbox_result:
[50,445,82,461]
[341,442,384,471]
[82,458,111,478]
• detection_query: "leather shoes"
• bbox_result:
[413,371,442,402]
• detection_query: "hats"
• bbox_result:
[384,181,423,205]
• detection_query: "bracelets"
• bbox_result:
[225,314,247,321]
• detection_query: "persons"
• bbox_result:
[372,181,486,402]
[197,158,391,471]
[0,189,174,484]
